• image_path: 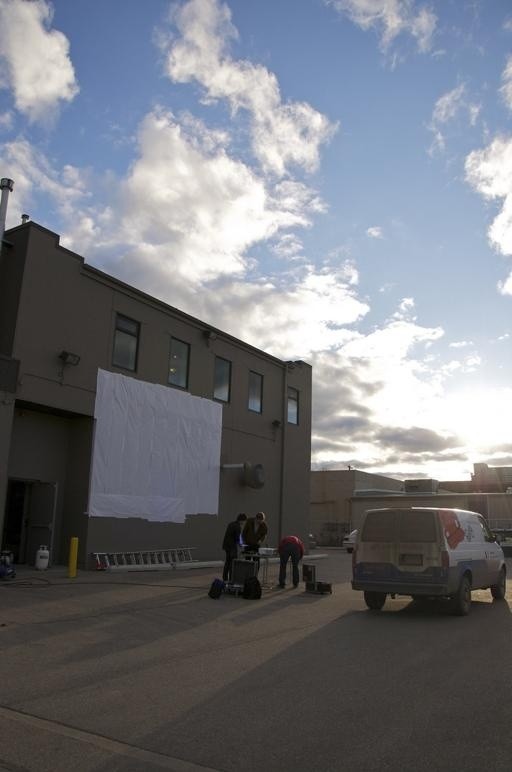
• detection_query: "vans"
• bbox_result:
[351,506,506,615]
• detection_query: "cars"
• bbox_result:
[342,529,357,553]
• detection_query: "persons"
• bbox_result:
[222,511,268,583]
[276,535,305,588]
